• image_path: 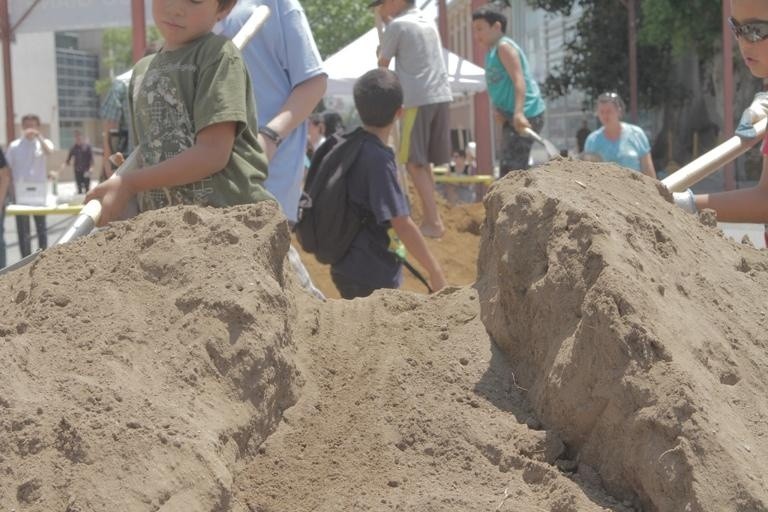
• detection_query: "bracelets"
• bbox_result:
[259,126,282,147]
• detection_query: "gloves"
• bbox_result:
[673,188,698,213]
[734,92,768,139]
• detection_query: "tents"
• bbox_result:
[323,24,486,96]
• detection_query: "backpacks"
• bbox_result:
[292,131,385,265]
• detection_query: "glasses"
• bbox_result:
[728,16,768,42]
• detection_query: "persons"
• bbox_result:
[4,115,55,258]
[83,1,279,228]
[212,1,324,299]
[472,9,546,177]
[98,41,162,185]
[366,0,454,238]
[670,0,768,250]
[330,69,447,298]
[65,128,95,194]
[448,141,477,176]
[583,91,657,179]
[1,148,10,268]
[576,120,591,153]
[303,110,348,179]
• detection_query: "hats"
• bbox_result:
[366,1,382,9]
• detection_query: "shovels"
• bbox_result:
[0,5,268,275]
[508,116,558,160]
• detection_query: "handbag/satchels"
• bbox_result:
[15,182,47,205]
